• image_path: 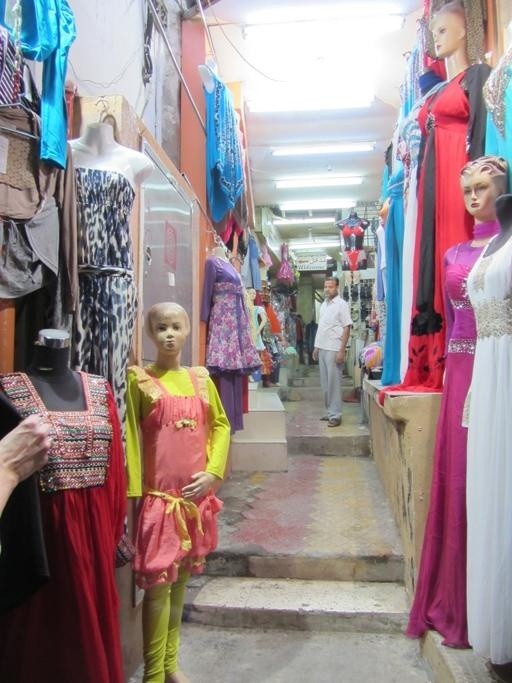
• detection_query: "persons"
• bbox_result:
[481,22,512,191]
[1,414,52,517]
[197,57,246,223]
[405,154,508,652]
[311,277,353,428]
[336,211,370,270]
[460,191,511,682]
[200,245,268,436]
[30,123,155,444]
[378,0,492,405]
[122,300,231,682]
[2,329,130,683]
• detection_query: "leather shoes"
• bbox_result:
[319,414,342,428]
